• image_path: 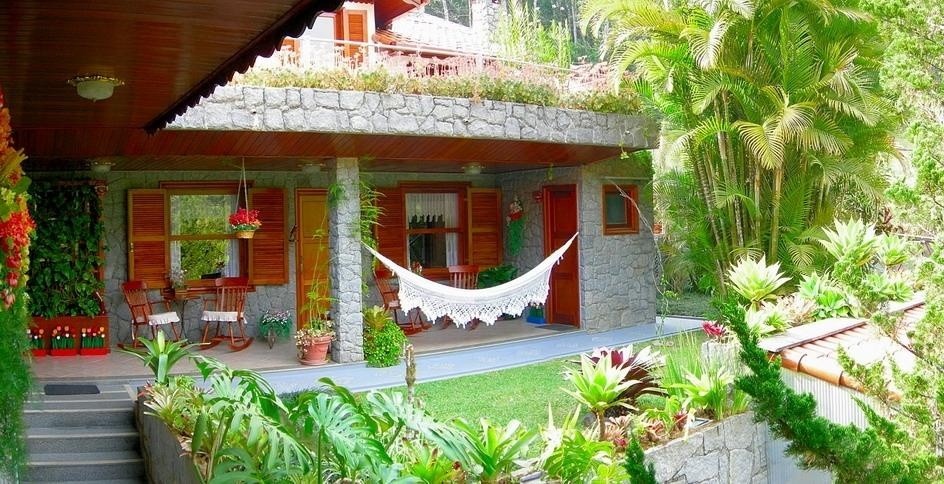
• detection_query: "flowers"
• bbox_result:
[258,310,292,337]
[295,320,336,360]
[227,206,262,231]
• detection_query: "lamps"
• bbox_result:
[460,160,487,176]
[84,160,116,173]
[296,161,326,174]
[64,72,125,104]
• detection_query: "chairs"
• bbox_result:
[115,273,255,352]
[371,263,481,335]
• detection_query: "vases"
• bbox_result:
[301,336,332,360]
